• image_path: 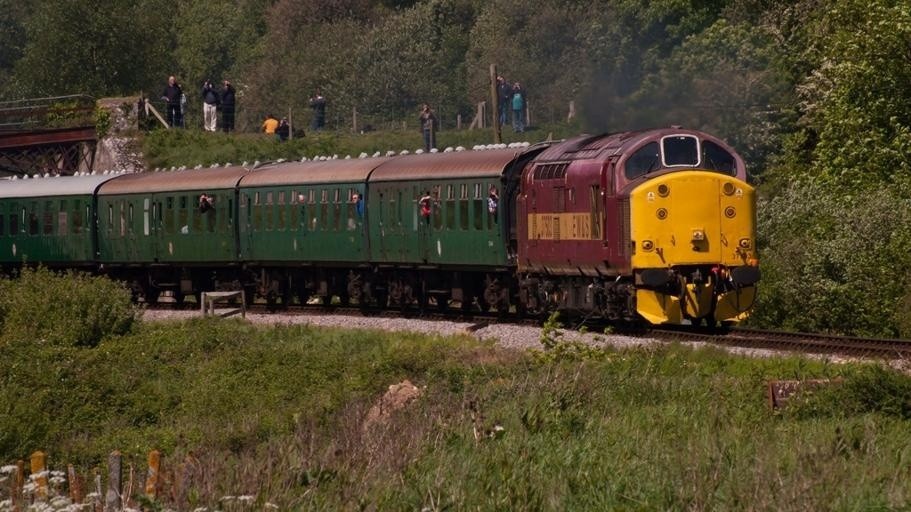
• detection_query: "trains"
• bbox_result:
[1,124,761,333]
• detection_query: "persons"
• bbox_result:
[496,75,512,126]
[308,91,327,130]
[488,184,500,214]
[418,194,431,218]
[351,194,364,216]
[199,194,215,232]
[298,193,305,204]
[416,102,438,153]
[510,81,525,132]
[160,75,294,139]
